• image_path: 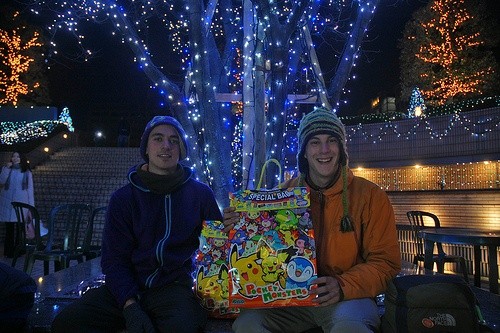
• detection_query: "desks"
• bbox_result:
[418,226,500,294]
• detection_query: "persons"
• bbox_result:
[223,108,401,333]
[50,116,233,333]
[0,151,34,263]
[0,260,38,333]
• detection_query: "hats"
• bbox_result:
[297,108,356,231]
[140,116,188,160]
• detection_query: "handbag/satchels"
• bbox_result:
[25,210,48,238]
[229,159,321,309]
[381,273,491,333]
[192,221,241,317]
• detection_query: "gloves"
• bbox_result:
[122,302,155,333]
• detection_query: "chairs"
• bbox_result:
[10,201,64,272]
[64,206,106,268]
[406,211,470,282]
[26,202,90,275]
